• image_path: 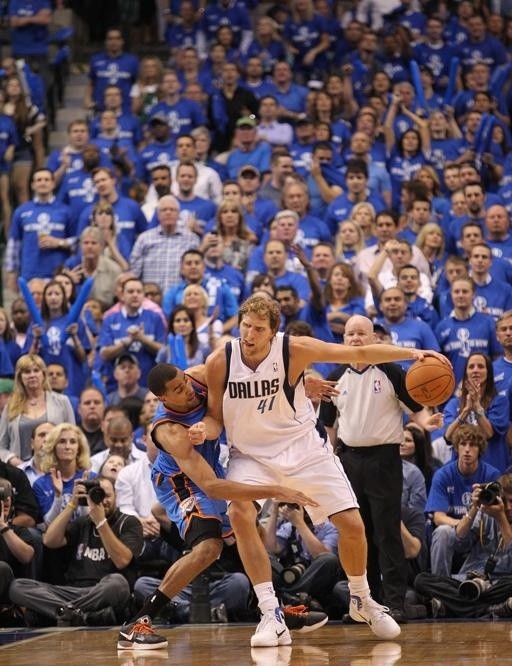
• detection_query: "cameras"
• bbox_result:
[0,487,9,516]
[279,539,311,589]
[78,480,106,507]
[476,482,503,506]
[279,502,300,510]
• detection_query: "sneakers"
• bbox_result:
[250,593,409,647]
[487,598,511,618]
[11,603,228,650]
[425,597,441,620]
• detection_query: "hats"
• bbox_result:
[237,117,256,127]
[114,354,139,366]
[150,114,167,124]
[294,112,314,124]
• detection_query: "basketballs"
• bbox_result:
[405,357,454,407]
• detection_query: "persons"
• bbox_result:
[115,361,342,651]
[184,287,452,647]
[0,0,511,623]
